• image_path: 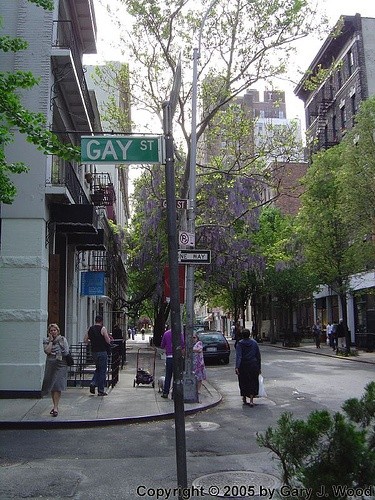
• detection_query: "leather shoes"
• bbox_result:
[318,346,320,349]
[197,390,201,394]
[50,409,54,414]
[317,346,318,349]
[53,410,58,417]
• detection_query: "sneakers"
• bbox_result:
[243,401,247,405]
[171,397,173,400]
[98,392,108,397]
[250,402,254,406]
[90,384,95,394]
[161,394,168,398]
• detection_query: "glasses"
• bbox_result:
[193,337,197,339]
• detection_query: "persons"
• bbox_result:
[141,327,145,340]
[327,322,333,347]
[83,316,113,396]
[134,325,138,334]
[127,326,131,338]
[233,323,244,349]
[131,328,136,340]
[337,319,346,350]
[161,329,186,399]
[41,323,69,417]
[234,329,263,409]
[313,319,323,348]
[231,324,235,332]
[332,320,339,352]
[191,333,206,397]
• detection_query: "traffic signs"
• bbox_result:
[80,135,166,164]
[160,199,187,209]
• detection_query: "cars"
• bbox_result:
[192,325,210,334]
[198,330,231,365]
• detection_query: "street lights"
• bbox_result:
[180,249,212,264]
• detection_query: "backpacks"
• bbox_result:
[142,330,144,335]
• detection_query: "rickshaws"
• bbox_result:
[133,347,157,388]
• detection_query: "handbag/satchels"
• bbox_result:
[232,326,242,341]
[255,374,267,398]
[313,326,320,337]
[57,342,74,367]
